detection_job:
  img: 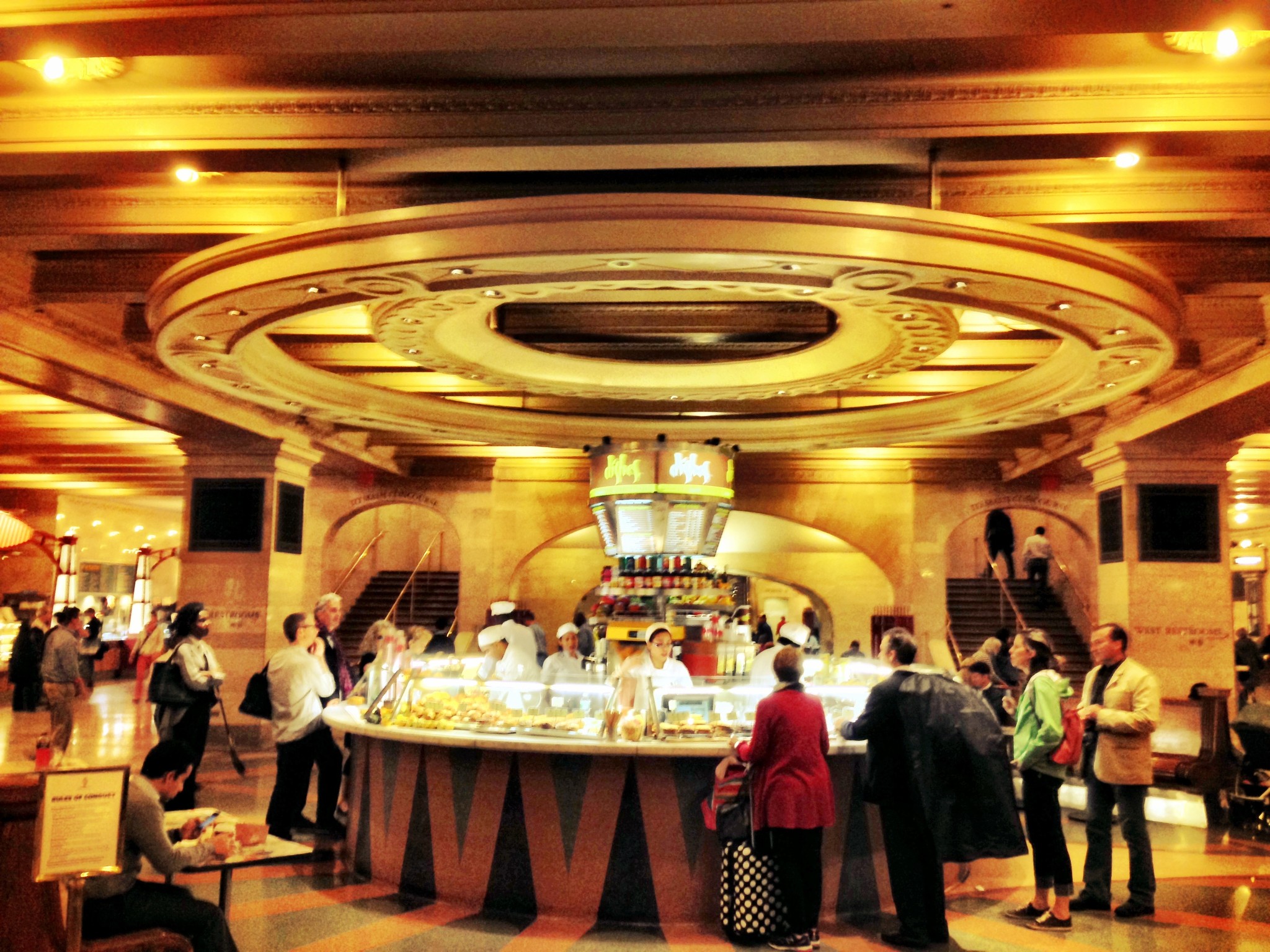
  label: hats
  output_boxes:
[490,600,515,616]
[477,624,505,651]
[556,622,579,639]
[779,622,811,645]
[645,621,671,643]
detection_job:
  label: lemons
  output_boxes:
[348,695,454,730]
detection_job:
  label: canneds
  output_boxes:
[609,556,707,588]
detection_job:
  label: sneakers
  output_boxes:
[810,929,821,946]
[1026,911,1073,930]
[1005,902,1050,918]
[767,932,812,951]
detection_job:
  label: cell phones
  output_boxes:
[197,810,221,829]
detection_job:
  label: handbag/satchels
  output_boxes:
[702,754,749,830]
[78,637,100,654]
[238,662,272,721]
[35,743,53,771]
[145,645,195,706]
[716,796,745,835]
[339,667,352,698]
[1052,698,1085,765]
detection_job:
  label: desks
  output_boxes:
[155,805,320,922]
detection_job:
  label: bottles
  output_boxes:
[624,558,633,587]
[718,563,729,589]
[645,556,661,588]
[634,557,646,588]
[680,557,693,588]
[671,557,680,588]
[35,733,51,770]
[610,558,624,588]
[662,557,671,587]
[693,562,714,589]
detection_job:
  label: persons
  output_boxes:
[981,508,1016,580]
[1188,622,1270,771]
[729,645,836,952]
[627,622,694,711]
[840,640,865,658]
[1022,526,1051,608]
[957,627,1025,745]
[0,592,609,952]
[835,627,950,952]
[1003,629,1075,931]
[1066,622,1161,919]
[724,605,823,684]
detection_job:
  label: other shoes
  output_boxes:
[879,929,948,951]
[1069,896,1110,913]
[1114,898,1155,918]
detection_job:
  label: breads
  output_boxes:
[620,715,754,742]
[418,690,584,732]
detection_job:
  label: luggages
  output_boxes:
[719,766,785,946]
